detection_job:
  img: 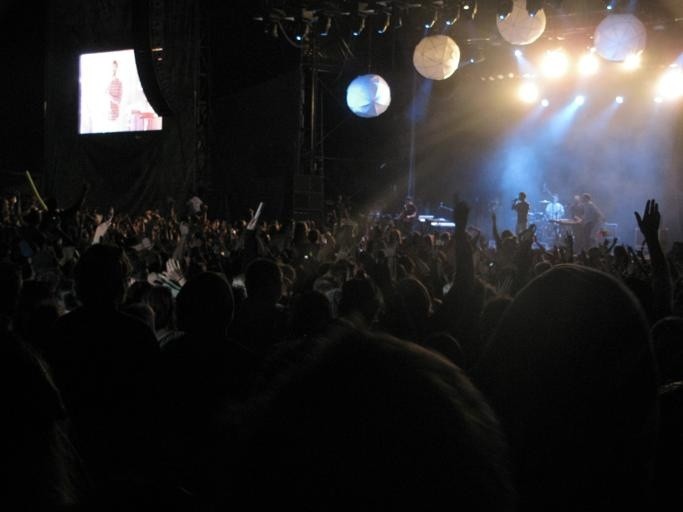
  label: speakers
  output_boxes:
[133,0,186,116]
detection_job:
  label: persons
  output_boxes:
[104,60,121,122]
[1,191,682,510]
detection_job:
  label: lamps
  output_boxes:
[594,12,648,62]
[412,34,460,82]
[346,24,391,118]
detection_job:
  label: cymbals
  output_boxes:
[538,200,551,204]
